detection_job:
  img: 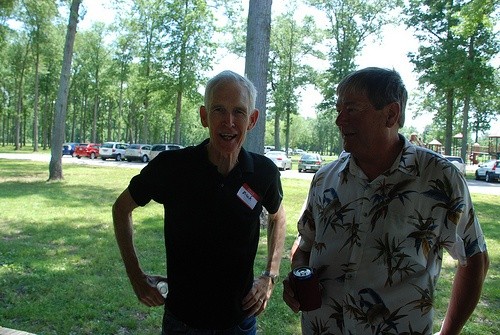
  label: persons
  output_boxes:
[283,67,491,335]
[111,70,287,335]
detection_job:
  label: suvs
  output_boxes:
[443,156,467,177]
[62,141,184,164]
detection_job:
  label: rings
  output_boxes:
[260,300,263,303]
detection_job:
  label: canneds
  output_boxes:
[292,266,313,279]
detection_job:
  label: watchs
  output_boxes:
[262,271,279,283]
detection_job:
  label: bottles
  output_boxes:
[156,282,168,298]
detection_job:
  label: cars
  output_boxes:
[298,154,325,173]
[264,151,293,171]
[475,160,500,182]
[264,146,304,155]
